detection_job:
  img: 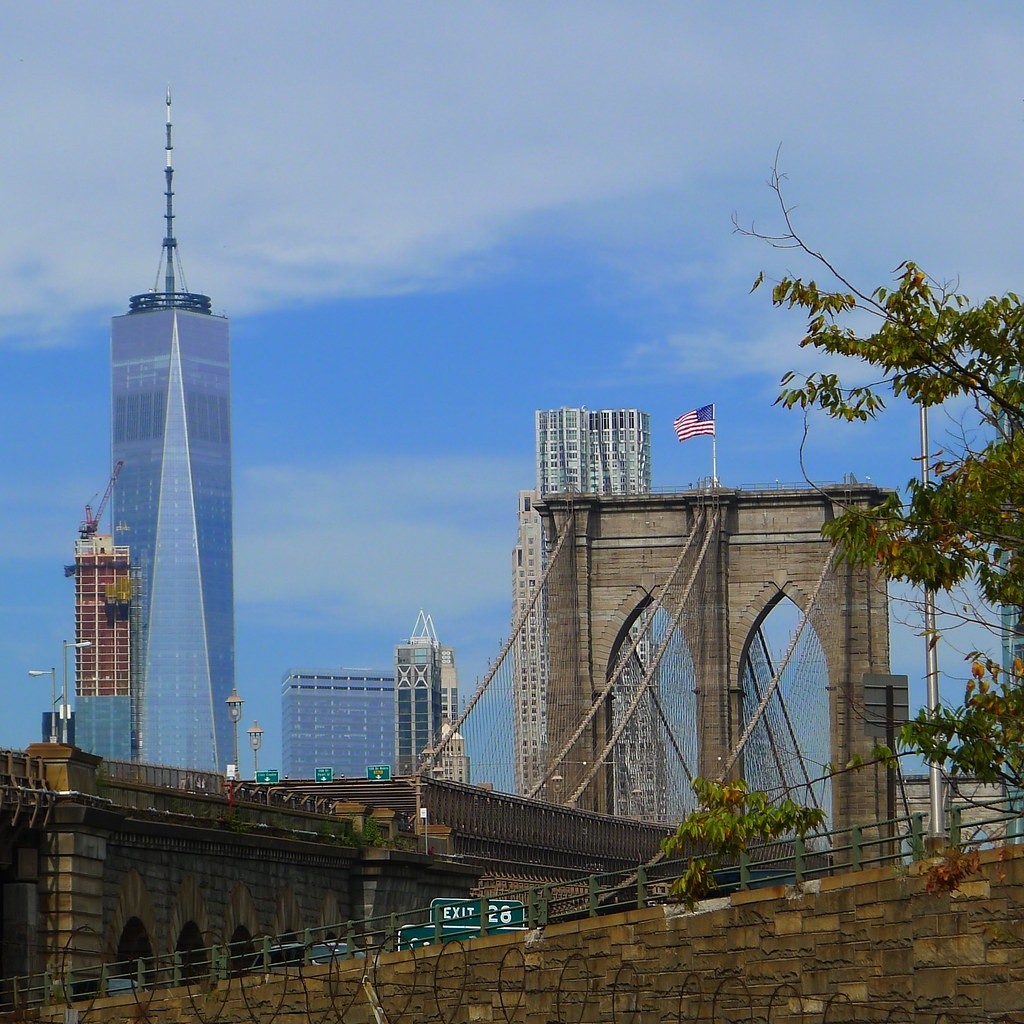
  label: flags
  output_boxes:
[673,403,714,442]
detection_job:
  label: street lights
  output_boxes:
[63,641,93,743]
[28,667,58,743]
[247,721,264,783]
[225,689,245,781]
[552,769,564,805]
